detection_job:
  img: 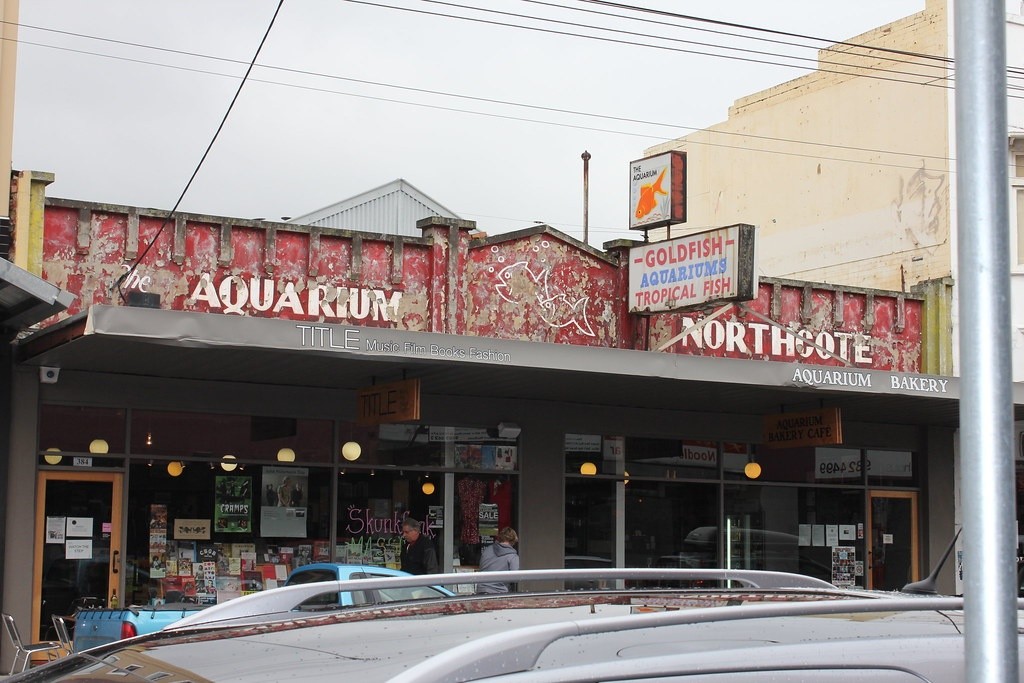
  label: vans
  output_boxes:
[680,526,815,589]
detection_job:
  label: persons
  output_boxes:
[400,519,437,575]
[477,528,520,594]
[205,580,217,594]
[873,528,885,580]
[281,555,290,564]
[197,565,204,579]
[153,556,159,567]
[181,560,190,571]
[266,476,302,507]
[153,541,165,548]
[151,519,166,529]
[299,550,310,559]
[249,579,262,590]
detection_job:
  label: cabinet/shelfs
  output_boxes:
[724,511,765,589]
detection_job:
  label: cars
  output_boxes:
[564,556,624,592]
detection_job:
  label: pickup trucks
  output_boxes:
[74,564,456,655]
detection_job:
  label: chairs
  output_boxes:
[0,612,74,677]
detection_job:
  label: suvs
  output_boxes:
[0,569,1024,683]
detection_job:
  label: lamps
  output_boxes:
[44,447,64,465]
[166,461,185,477]
[220,455,238,471]
[277,448,296,463]
[623,472,630,485]
[580,462,597,476]
[88,439,110,454]
[422,482,435,496]
[744,462,763,480]
[342,441,362,461]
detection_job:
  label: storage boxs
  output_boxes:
[454,444,518,471]
